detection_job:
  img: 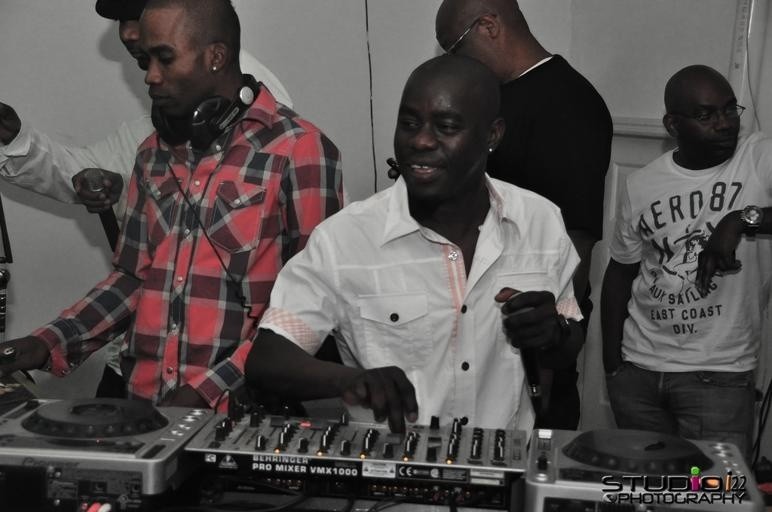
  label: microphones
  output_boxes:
[84,169,121,253]
[507,292,543,398]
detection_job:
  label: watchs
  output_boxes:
[743,205,760,238]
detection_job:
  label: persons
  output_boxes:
[245,54,580,429]
[600,66,772,465]
[0,0,344,416]
[0,0,293,398]
[388,0,613,344]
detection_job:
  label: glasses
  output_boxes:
[667,104,746,124]
[441,13,497,54]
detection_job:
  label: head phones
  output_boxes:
[151,73,261,155]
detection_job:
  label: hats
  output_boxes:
[95,1,147,20]
